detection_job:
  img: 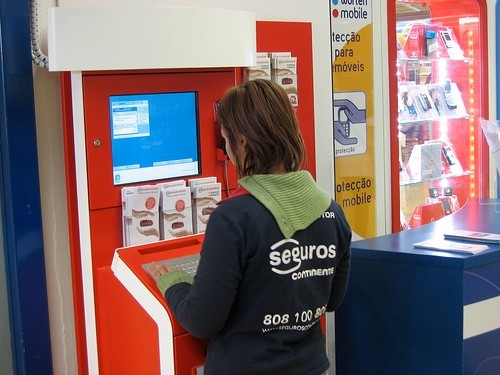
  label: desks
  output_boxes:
[335,196,500,375]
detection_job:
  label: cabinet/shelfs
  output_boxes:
[396,55,475,230]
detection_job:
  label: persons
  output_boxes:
[146,77,353,375]
[402,89,444,120]
